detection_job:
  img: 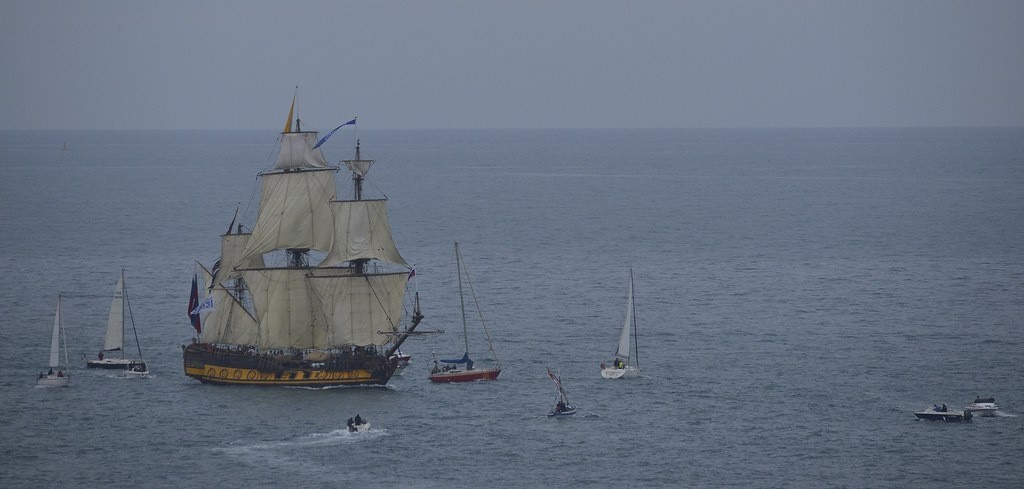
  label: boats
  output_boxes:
[914,405,960,421]
[965,398,998,417]
[347,417,371,435]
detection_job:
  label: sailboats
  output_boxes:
[183,84,423,387]
[600,264,641,379]
[547,366,576,417]
[86,268,146,372]
[35,295,70,386]
[426,241,502,383]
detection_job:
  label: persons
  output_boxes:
[98,350,104,361]
[354,414,362,426]
[347,416,354,431]
[38,369,64,379]
[614,358,623,369]
